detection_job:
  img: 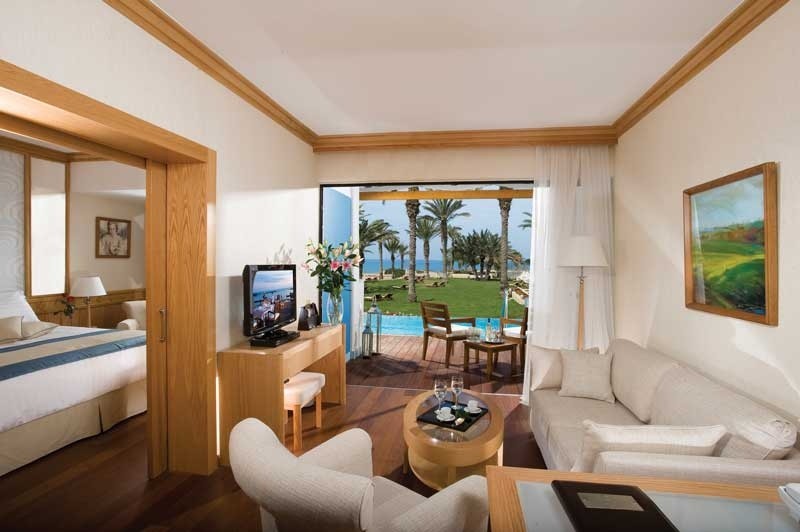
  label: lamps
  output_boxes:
[70,277,108,326]
[557,234,609,350]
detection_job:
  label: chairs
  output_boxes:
[228,417,490,532]
[496,305,528,374]
[420,301,475,367]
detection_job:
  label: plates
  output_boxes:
[464,407,482,414]
[436,414,456,421]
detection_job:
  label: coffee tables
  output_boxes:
[403,387,503,490]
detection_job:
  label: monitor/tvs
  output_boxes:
[243,265,300,347]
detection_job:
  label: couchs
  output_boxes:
[529,338,800,476]
[116,300,147,332]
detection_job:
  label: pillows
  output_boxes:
[570,418,725,472]
[559,351,616,403]
[0,316,25,342]
[22,321,58,339]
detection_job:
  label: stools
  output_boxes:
[285,371,327,450]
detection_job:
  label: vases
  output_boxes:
[327,288,344,326]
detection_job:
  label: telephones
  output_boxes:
[778,483,800,522]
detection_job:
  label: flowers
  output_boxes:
[55,293,76,320]
[303,239,364,292]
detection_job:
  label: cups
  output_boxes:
[438,407,451,418]
[468,400,479,411]
[466,327,482,341]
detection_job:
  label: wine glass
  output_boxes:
[433,378,448,414]
[451,376,463,410]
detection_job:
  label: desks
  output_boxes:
[465,338,517,379]
[217,322,346,467]
[486,465,800,532]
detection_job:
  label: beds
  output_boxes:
[0,291,147,478]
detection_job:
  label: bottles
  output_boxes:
[486,317,492,341]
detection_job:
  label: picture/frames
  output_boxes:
[681,161,781,325]
[95,217,132,261]
